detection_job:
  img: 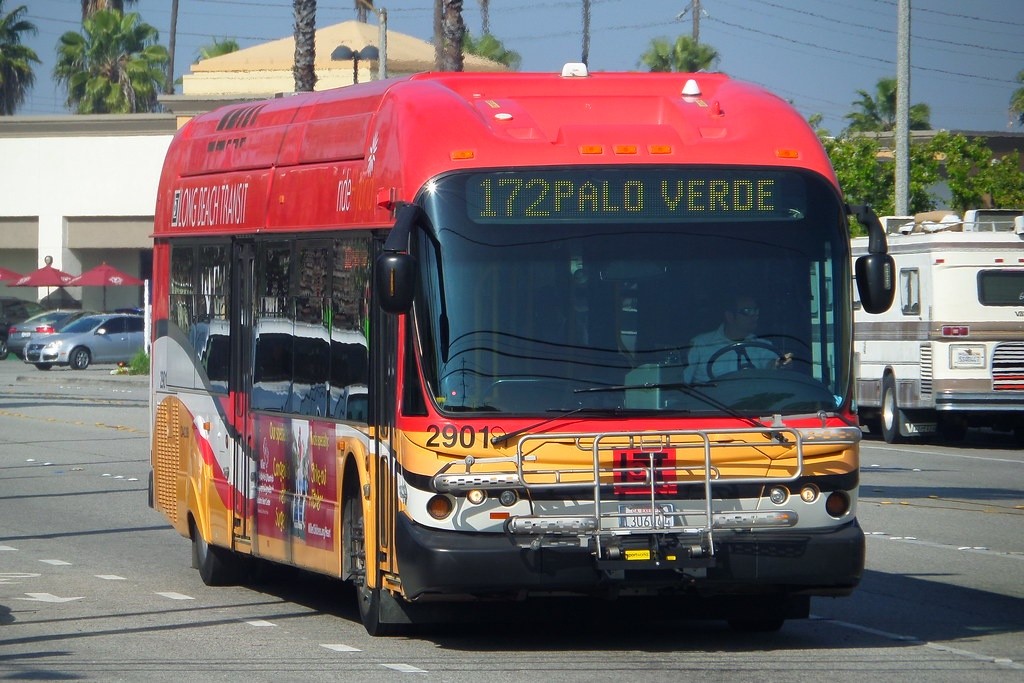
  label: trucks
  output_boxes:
[843,207,1024,445]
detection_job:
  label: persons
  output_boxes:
[683,286,795,385]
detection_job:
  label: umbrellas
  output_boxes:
[0,255,144,308]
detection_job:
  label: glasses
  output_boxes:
[733,308,760,317]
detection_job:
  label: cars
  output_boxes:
[22,313,147,373]
[8,309,100,361]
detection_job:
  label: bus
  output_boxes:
[146,61,898,642]
